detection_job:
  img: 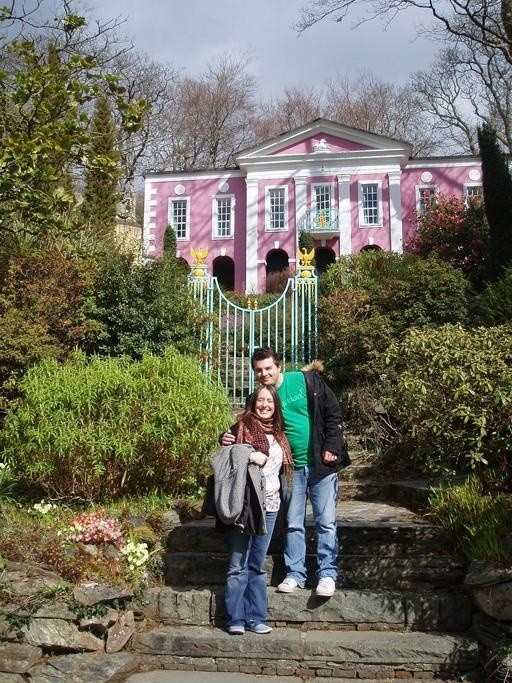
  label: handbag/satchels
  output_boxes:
[201,474,217,515]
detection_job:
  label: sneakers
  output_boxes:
[277,577,299,592]
[315,577,335,596]
[228,624,273,634]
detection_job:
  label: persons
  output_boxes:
[219,348,352,597]
[223,384,293,634]
